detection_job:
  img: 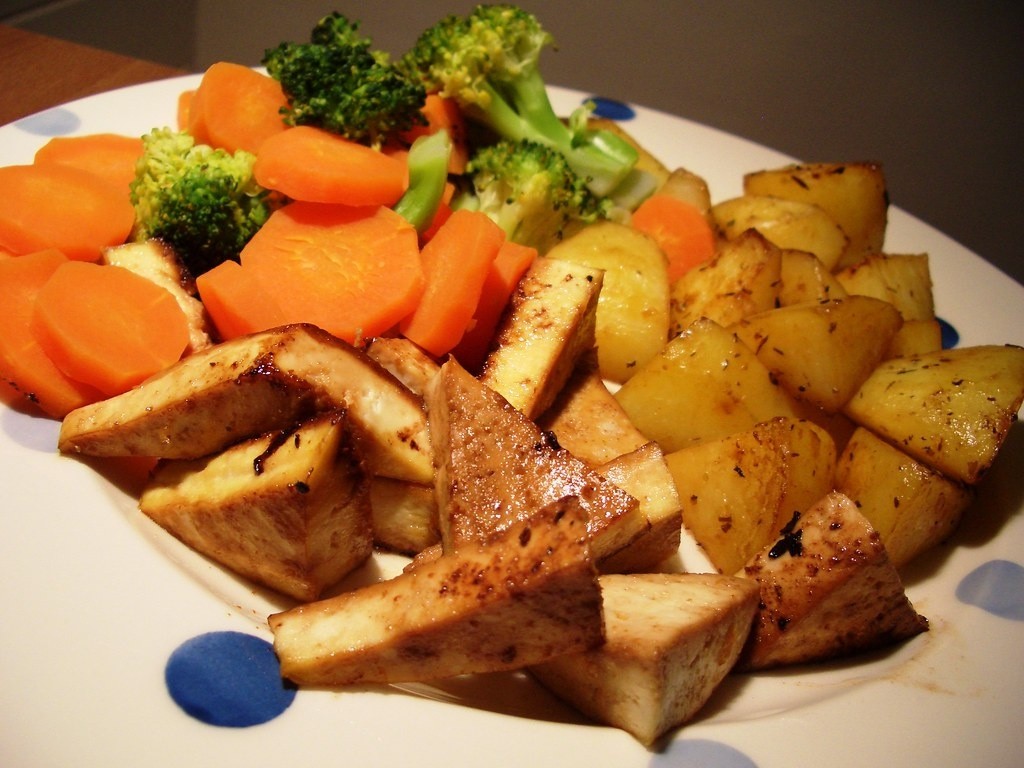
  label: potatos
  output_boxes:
[545,158,1024,575]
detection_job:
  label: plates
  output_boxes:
[0,66,1023,768]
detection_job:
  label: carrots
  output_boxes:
[0,61,713,414]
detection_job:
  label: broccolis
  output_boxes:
[127,3,641,273]
